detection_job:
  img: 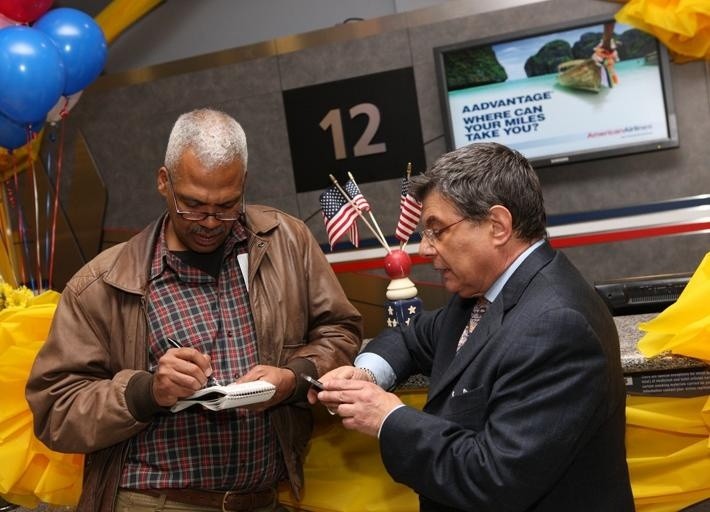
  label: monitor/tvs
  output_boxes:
[432,12,680,171]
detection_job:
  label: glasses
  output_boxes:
[167,171,248,223]
[417,209,485,240]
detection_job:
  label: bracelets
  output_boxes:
[358,367,376,386]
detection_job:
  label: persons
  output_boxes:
[308,140,636,512]
[24,107,364,512]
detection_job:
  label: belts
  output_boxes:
[128,485,280,512]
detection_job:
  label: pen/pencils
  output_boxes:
[167,338,223,387]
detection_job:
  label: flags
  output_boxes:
[345,178,371,249]
[394,176,422,244]
[319,184,362,252]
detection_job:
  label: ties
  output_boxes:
[456,301,488,352]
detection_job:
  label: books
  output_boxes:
[169,378,277,414]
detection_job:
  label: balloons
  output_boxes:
[0,0,110,156]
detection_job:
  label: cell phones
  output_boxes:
[299,372,324,391]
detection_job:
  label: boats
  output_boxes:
[645,52,658,65]
[557,23,617,94]
